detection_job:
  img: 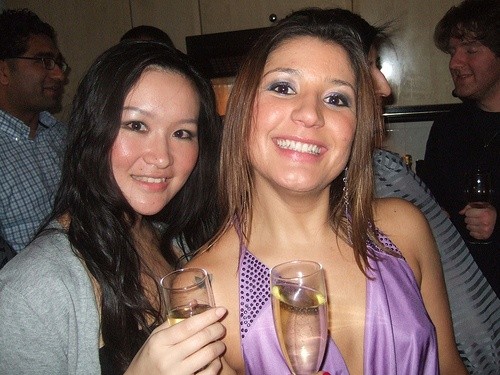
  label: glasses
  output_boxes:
[0,53,68,73]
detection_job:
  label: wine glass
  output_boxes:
[460,166,497,246]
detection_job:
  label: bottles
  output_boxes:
[402,153,413,167]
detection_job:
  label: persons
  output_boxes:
[331,8,500,375]
[423,0,500,301]
[0,40,228,375]
[0,8,68,269]
[171,16,466,375]
[120,25,176,51]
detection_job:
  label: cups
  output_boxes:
[159,266,216,328]
[269,258,331,375]
